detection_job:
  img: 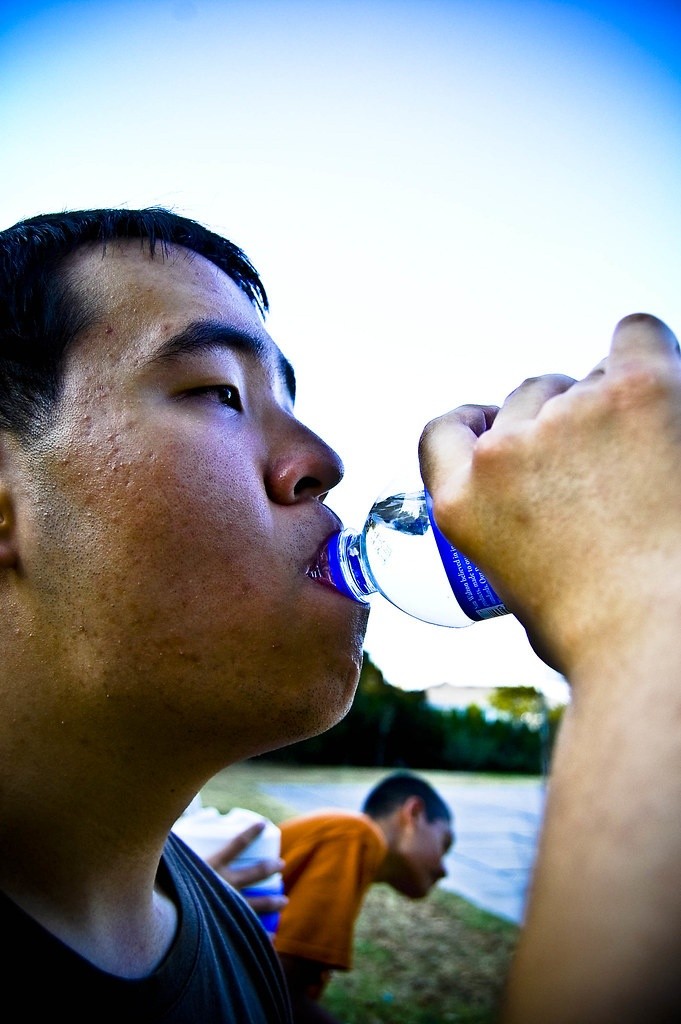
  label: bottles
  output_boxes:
[306,484,514,629]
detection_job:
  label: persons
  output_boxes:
[210,823,289,914]
[1,208,681,1024]
[273,772,454,1006]
